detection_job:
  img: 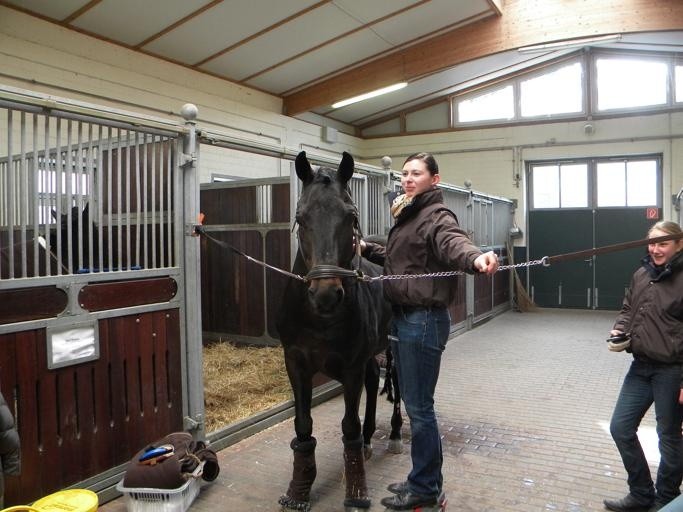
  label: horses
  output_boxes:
[50,201,124,275]
[276,151,403,511]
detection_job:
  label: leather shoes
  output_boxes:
[381,490,440,510]
[603,490,658,512]
[386,478,443,495]
[647,488,682,512]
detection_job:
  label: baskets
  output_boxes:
[115,456,206,512]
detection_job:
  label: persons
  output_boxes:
[348,150,501,509]
[600,220,683,511]
